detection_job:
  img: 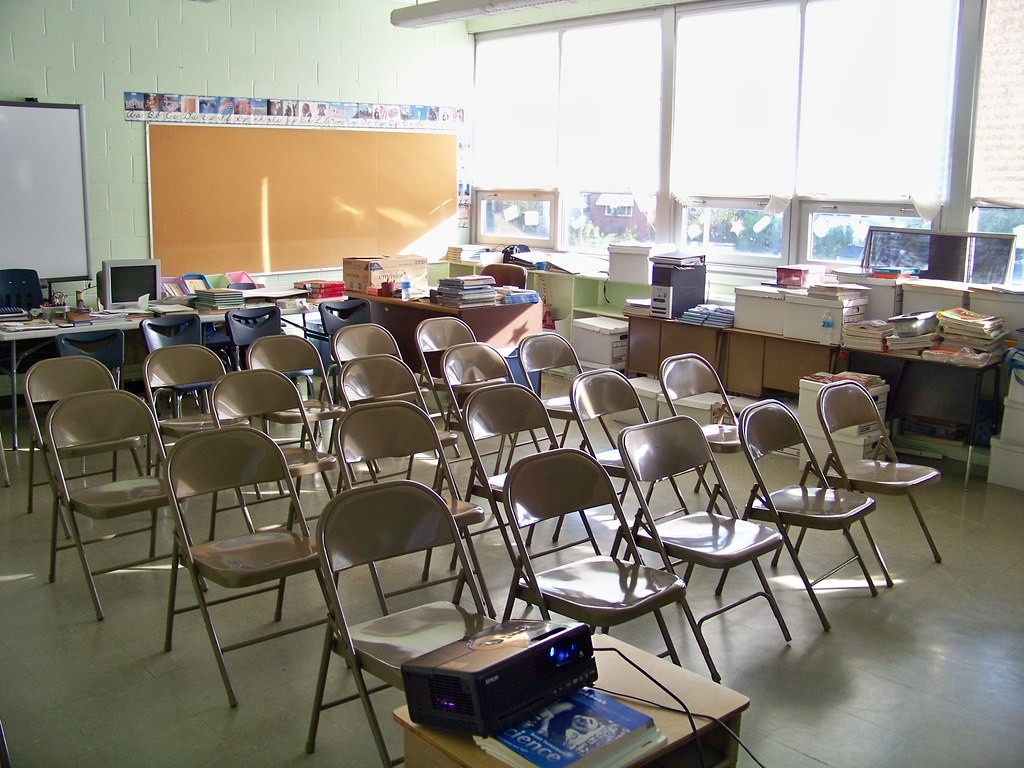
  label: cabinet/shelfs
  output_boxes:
[720,327,839,399]
[627,312,720,376]
[448,260,652,376]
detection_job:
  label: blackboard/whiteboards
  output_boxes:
[0,98,93,288]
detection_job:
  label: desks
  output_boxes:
[393,635,747,767]
[845,347,1003,493]
[0,289,543,450]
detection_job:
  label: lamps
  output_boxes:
[390,0,553,31]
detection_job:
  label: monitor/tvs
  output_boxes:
[95,260,162,311]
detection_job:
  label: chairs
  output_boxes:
[25,296,946,767]
[481,263,528,291]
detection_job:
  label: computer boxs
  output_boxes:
[650,267,706,320]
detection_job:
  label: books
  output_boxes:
[65,243,1010,352]
[474,686,668,768]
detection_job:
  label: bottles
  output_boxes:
[819,310,833,345]
[401,271,411,301]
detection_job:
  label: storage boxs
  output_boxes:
[987,366,1024,491]
[572,316,625,362]
[734,286,785,337]
[769,398,799,459]
[797,378,890,436]
[341,255,427,292]
[799,430,890,481]
[713,397,759,444]
[656,389,732,435]
[785,294,867,342]
[611,377,662,426]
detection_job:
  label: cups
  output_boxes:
[381,282,395,297]
